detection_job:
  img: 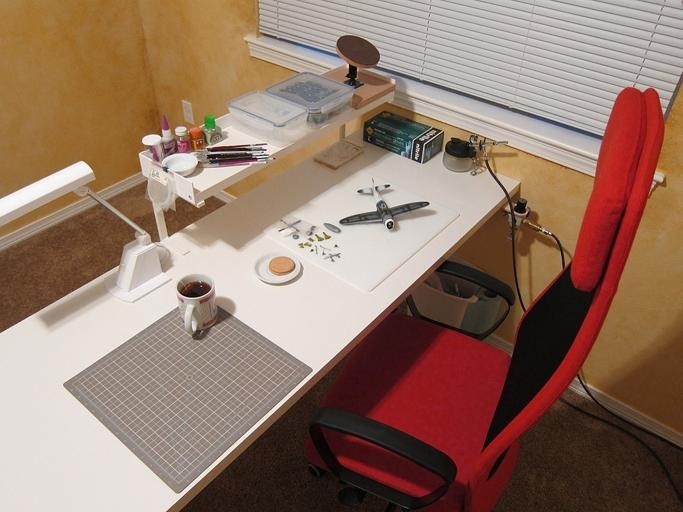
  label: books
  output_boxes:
[321,64,395,109]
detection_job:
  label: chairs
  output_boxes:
[307,86,663,511]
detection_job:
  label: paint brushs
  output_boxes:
[207,143,276,166]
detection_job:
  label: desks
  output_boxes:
[0,65,523,510]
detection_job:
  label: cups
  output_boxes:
[174,273,218,334]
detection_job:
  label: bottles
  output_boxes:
[443,137,476,174]
[142,113,223,164]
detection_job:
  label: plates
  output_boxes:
[253,252,303,285]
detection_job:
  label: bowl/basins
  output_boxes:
[162,153,198,176]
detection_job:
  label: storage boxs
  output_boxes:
[262,71,355,132]
[225,90,307,139]
